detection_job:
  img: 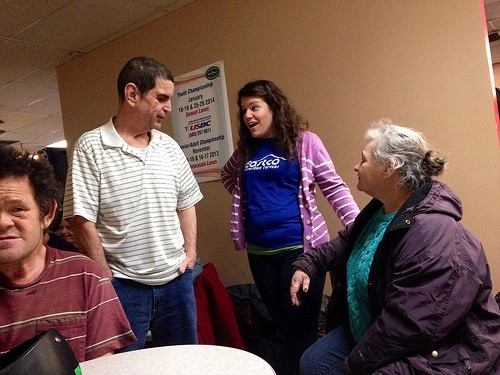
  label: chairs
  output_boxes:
[193,262,218,328]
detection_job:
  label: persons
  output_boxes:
[63,56,204,352]
[291,119,500,375]
[220,80,363,375]
[0,144,140,363]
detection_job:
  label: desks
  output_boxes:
[78,344,276,375]
